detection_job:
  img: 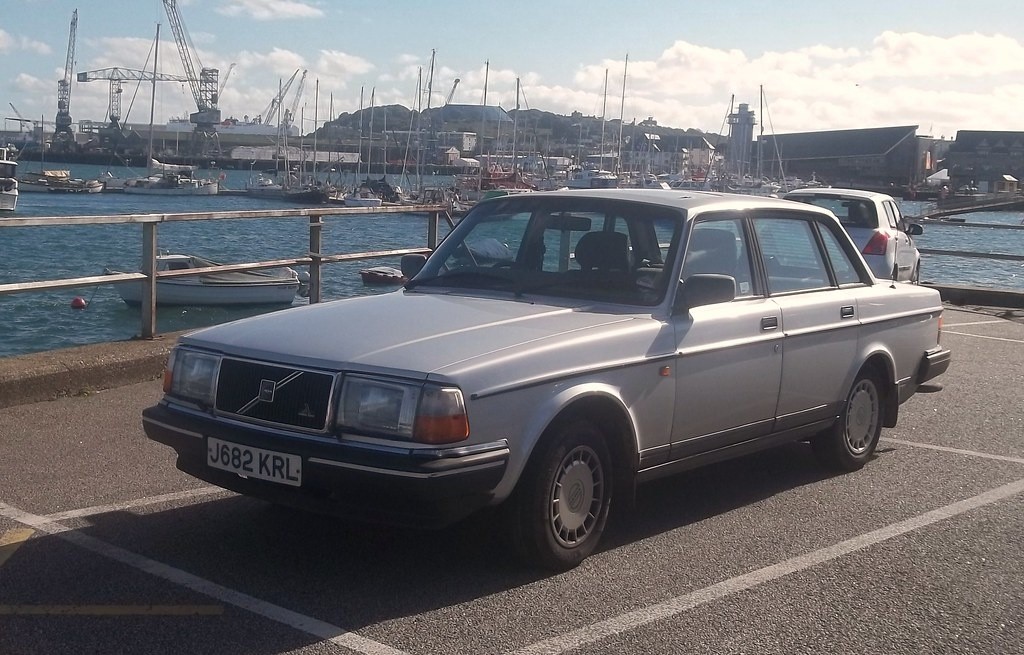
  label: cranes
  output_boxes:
[283,69,308,125]
[49,8,78,153]
[76,67,195,152]
[161,0,223,157]
[257,68,301,125]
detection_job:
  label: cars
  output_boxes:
[765,187,924,286]
[139,187,953,574]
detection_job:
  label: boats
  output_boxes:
[101,254,301,305]
[0,49,821,212]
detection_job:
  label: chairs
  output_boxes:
[574,231,632,291]
[682,228,736,280]
[848,201,874,225]
